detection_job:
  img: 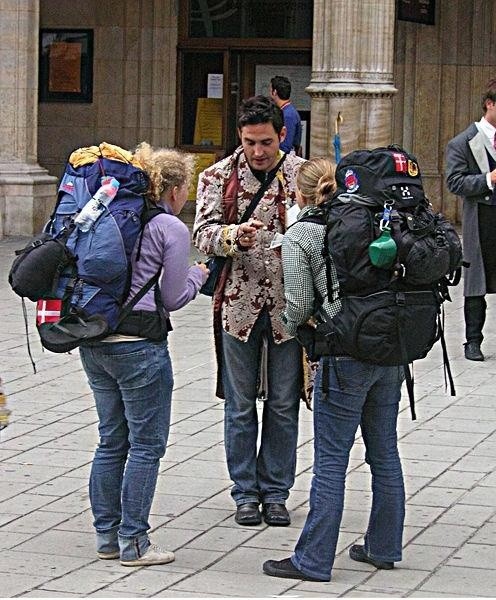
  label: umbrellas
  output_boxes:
[333,116,343,164]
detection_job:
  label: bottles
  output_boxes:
[72,179,120,233]
[369,227,397,270]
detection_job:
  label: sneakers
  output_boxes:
[97,543,175,566]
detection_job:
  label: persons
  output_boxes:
[192,95,309,527]
[268,76,302,155]
[263,157,406,582]
[78,142,211,567]
[445,79,496,361]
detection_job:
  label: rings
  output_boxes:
[245,236,249,242]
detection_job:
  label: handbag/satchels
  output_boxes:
[199,252,228,295]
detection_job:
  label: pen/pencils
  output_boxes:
[233,224,254,245]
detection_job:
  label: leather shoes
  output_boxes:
[465,341,484,361]
[235,501,290,526]
[263,558,330,581]
[349,545,394,569]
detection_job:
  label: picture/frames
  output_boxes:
[37,27,95,104]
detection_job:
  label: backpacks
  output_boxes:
[303,144,470,419]
[8,142,165,374]
